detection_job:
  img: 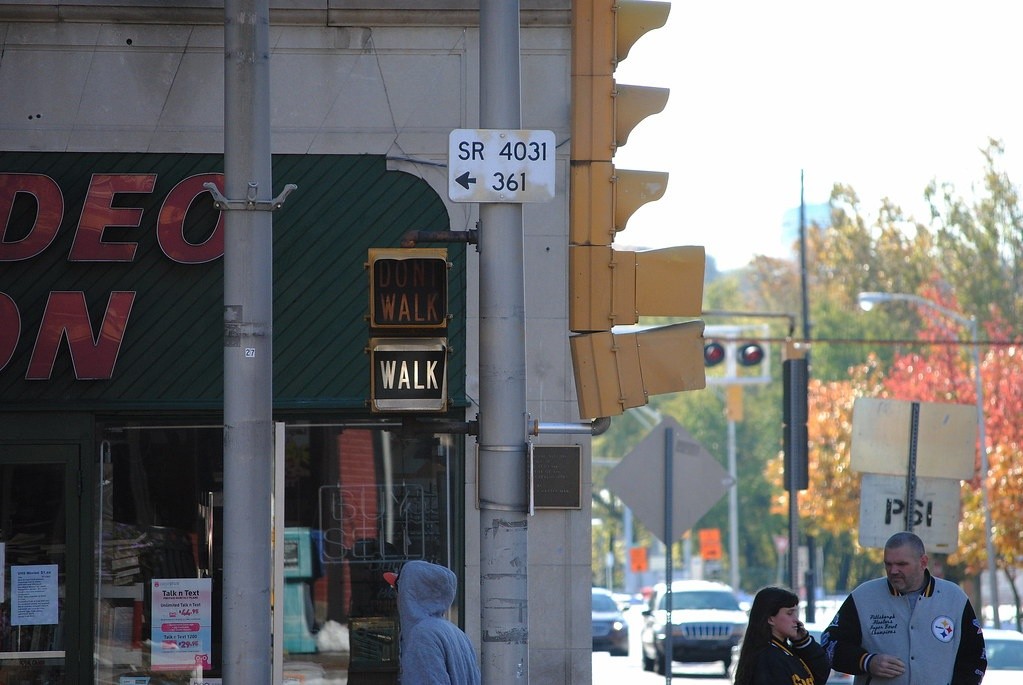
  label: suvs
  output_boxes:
[640,580,750,675]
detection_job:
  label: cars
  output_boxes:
[980,628,1023,685]
[728,625,854,685]
[590,587,630,656]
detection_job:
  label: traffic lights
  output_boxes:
[702,325,771,382]
[567,0,708,420]
[363,247,449,413]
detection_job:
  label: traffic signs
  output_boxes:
[448,128,557,204]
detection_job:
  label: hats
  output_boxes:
[383,560,406,594]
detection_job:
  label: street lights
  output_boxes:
[857,292,1000,630]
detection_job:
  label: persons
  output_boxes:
[733,587,831,685]
[821,532,988,685]
[383,560,482,685]
